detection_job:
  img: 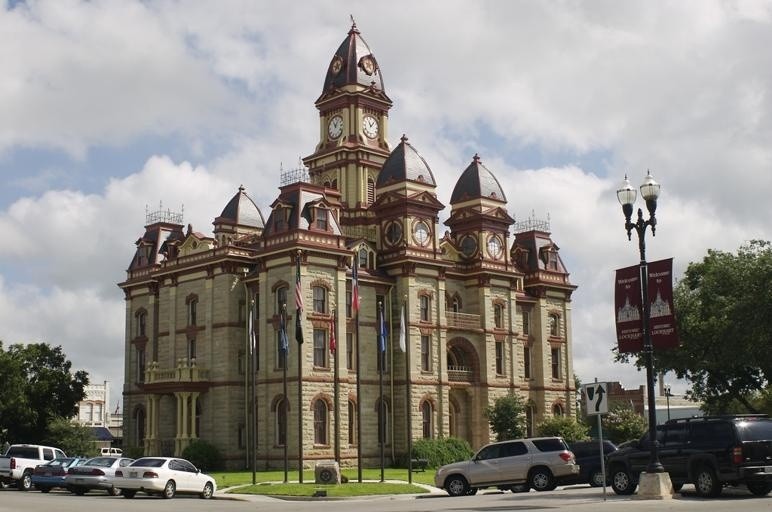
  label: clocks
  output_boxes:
[363,114,380,139]
[327,115,343,140]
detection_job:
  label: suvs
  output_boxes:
[605,411,772,501]
[511,438,620,493]
[436,436,581,496]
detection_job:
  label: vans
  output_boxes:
[96,447,124,459]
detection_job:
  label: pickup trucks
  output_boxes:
[0,444,68,492]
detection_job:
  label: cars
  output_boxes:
[112,456,217,498]
[65,454,135,496]
[30,456,87,495]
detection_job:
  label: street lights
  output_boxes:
[616,168,669,475]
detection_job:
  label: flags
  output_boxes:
[247,308,257,355]
[351,253,360,314]
[293,259,304,316]
[399,305,406,352]
[278,308,289,352]
[329,312,336,354]
[379,312,386,354]
